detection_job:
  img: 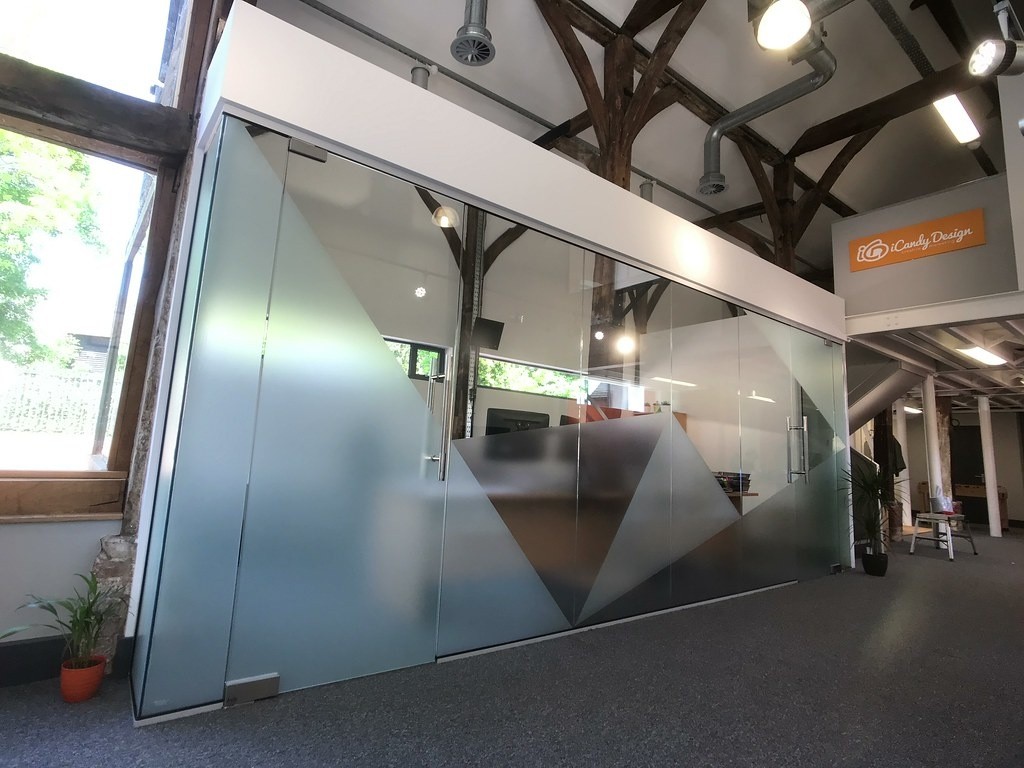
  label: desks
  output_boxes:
[910,513,977,561]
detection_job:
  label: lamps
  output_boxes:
[968,0,1024,78]
[754,0,811,50]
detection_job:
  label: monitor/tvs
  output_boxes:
[470,317,505,350]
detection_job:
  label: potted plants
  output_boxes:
[0,573,134,703]
[836,455,912,577]
